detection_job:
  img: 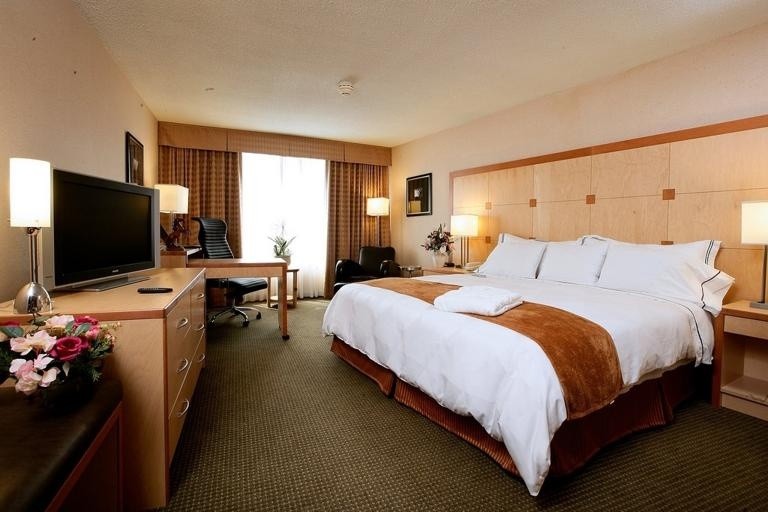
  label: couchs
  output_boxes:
[333,246,401,294]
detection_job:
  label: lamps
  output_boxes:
[741,200,768,310]
[154,184,189,244]
[9,158,51,313]
[450,215,478,267]
[366,197,390,247]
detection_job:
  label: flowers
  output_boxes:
[420,223,456,256]
[0,314,120,395]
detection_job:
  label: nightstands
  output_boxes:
[712,300,768,421]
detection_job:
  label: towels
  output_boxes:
[434,285,523,316]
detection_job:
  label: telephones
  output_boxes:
[465,262,480,271]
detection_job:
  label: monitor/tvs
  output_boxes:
[37,167,160,292]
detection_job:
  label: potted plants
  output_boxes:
[268,225,297,266]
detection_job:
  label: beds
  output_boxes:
[322,271,714,496]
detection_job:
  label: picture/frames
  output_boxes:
[406,173,432,217]
[125,131,144,185]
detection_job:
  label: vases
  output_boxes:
[433,250,446,268]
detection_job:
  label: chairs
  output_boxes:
[191,217,268,327]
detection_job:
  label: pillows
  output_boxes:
[474,232,721,304]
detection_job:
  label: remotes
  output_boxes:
[137,287,173,293]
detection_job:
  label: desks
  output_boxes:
[0,268,207,506]
[1,378,129,511]
[187,258,289,340]
